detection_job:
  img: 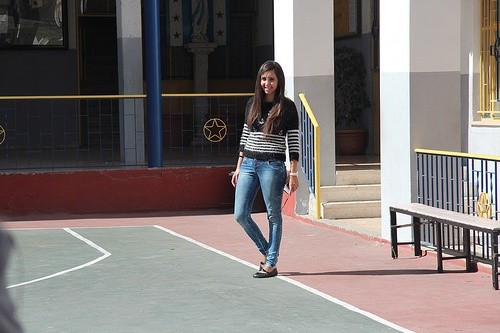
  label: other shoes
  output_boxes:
[252,259,279,278]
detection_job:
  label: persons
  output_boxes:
[231,61,300,278]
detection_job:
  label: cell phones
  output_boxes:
[284,183,290,196]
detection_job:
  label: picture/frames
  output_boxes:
[0,0,69,51]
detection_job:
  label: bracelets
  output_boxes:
[238,156,243,158]
[288,172,298,177]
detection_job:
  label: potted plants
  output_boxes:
[334,44,372,156]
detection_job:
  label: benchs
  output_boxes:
[389,203,500,290]
